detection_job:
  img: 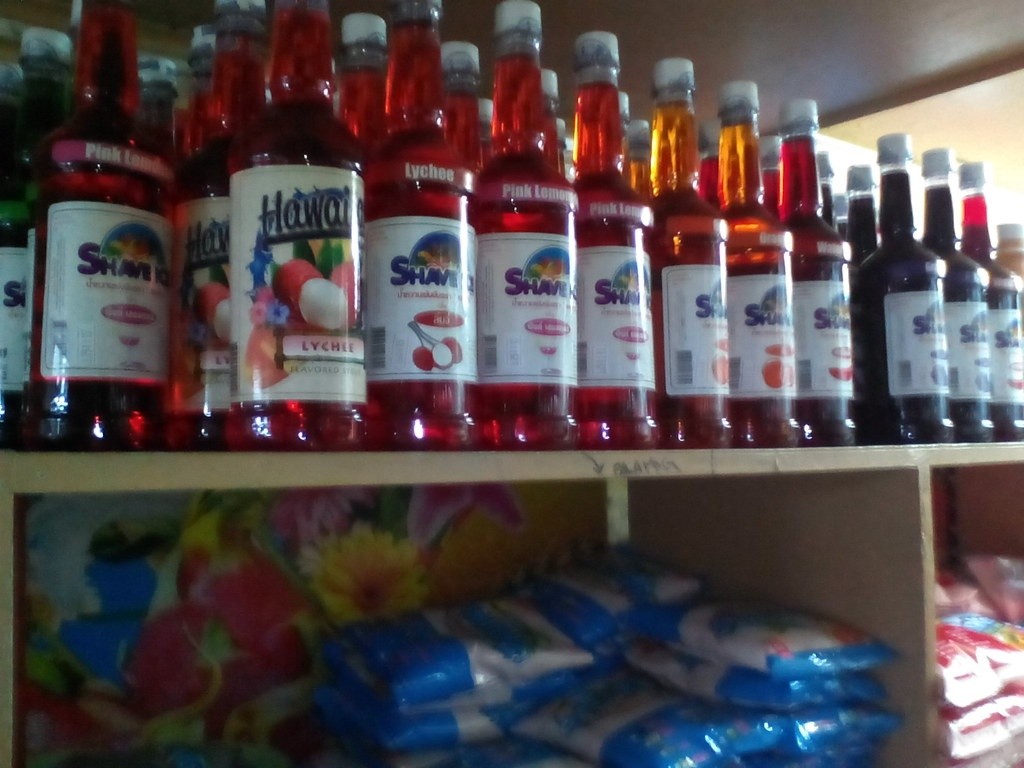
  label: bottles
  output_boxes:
[0,0,1023,449]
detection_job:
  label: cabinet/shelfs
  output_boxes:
[0,441,1024,768]
